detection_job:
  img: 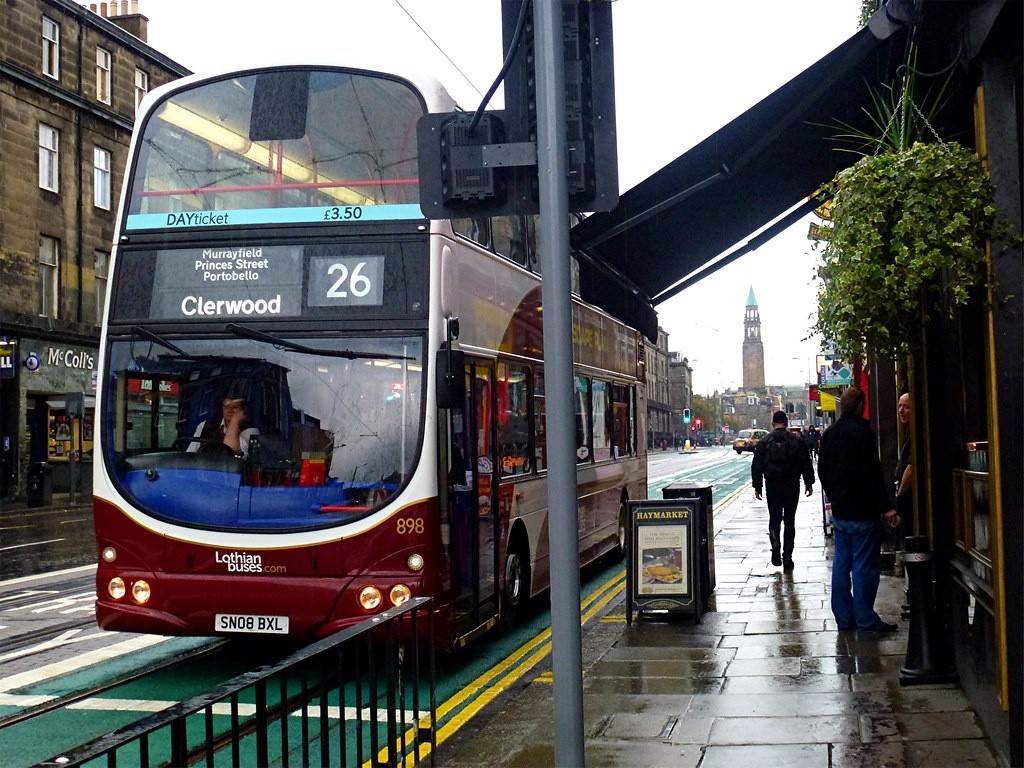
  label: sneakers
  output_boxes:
[784,558,794,570]
[771,543,781,566]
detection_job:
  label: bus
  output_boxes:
[93,58,649,656]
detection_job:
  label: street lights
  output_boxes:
[791,357,817,426]
[706,372,735,446]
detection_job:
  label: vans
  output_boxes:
[733,429,770,454]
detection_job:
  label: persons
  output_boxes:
[751,411,816,571]
[186,388,264,483]
[645,417,829,459]
[886,391,945,615]
[816,386,899,636]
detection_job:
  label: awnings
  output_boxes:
[565,0,946,348]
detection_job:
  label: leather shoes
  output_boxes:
[901,611,911,619]
[901,604,911,610]
[839,622,899,632]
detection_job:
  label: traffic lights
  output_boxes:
[696,418,702,430]
[691,425,696,431]
[683,409,691,424]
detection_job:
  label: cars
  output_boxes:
[786,426,824,448]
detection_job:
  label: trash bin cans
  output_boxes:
[26,462,53,508]
[661,480,716,597]
